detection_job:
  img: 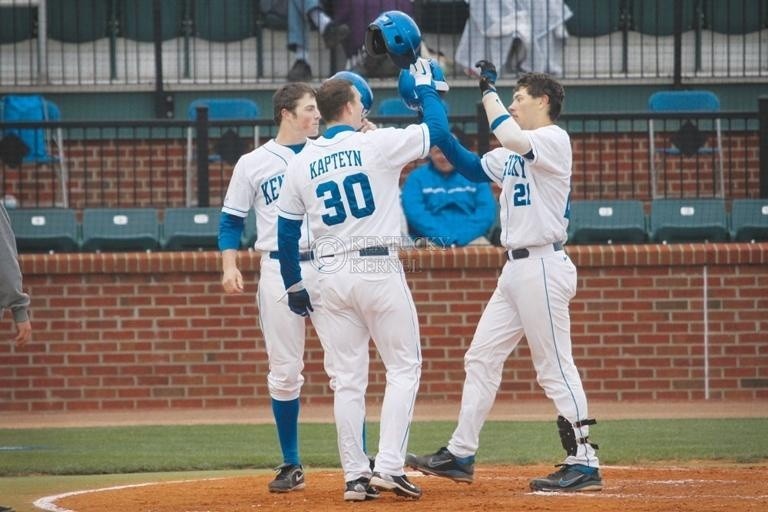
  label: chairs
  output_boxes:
[643,84,727,198]
[4,196,766,254]
[180,97,262,209]
[0,0,768,47]
[0,92,72,207]
[374,94,449,189]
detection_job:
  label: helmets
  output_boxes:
[398,55,449,112]
[363,9,422,63]
[330,71,373,111]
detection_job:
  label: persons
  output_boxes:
[401,59,604,491]
[216,83,368,492]
[261,0,350,81]
[275,60,449,500]
[401,131,498,246]
[0,198,33,349]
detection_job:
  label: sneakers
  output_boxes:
[287,59,311,83]
[528,463,601,492]
[322,21,349,49]
[404,447,474,483]
[368,468,424,502]
[344,476,380,503]
[269,462,307,494]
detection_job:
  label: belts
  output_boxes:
[269,251,317,261]
[321,245,387,257]
[504,241,564,260]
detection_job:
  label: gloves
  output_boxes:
[409,58,433,87]
[476,59,496,93]
[287,289,314,318]
